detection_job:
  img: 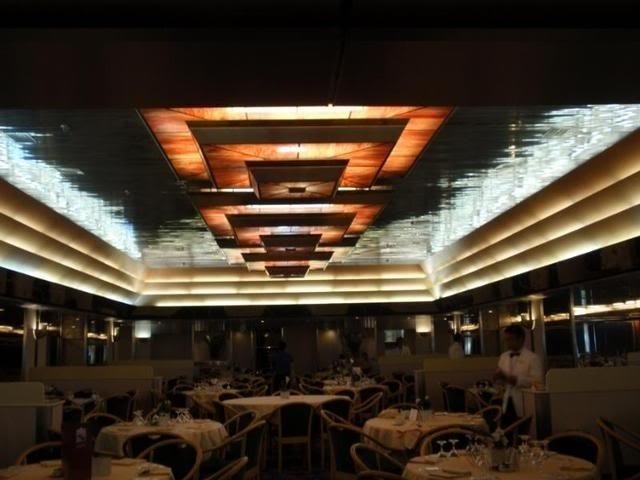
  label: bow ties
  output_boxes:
[509,352,520,358]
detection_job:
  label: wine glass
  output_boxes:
[437,435,550,469]
[192,378,232,393]
[133,408,194,427]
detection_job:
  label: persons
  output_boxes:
[498,325,543,447]
[272,341,295,391]
[394,337,412,356]
[450,334,464,359]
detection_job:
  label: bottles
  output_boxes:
[414,394,432,422]
[474,445,490,480]
[472,379,489,393]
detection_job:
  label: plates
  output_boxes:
[116,421,133,431]
[379,412,486,425]
[424,456,464,477]
